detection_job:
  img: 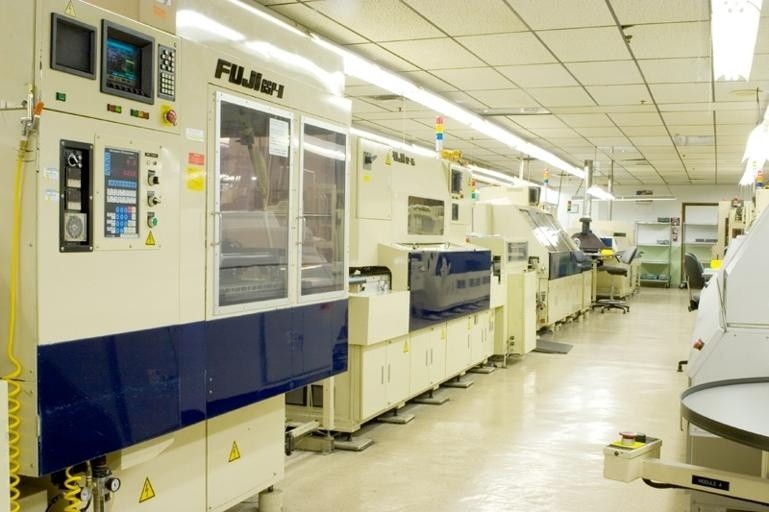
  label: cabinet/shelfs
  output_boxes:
[207,80,354,319]
[632,218,674,290]
[715,187,769,264]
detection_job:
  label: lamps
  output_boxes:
[705,0,764,82]
[566,169,679,202]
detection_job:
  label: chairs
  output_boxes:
[591,244,639,314]
[683,250,710,313]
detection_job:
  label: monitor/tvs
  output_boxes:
[106,35,141,89]
